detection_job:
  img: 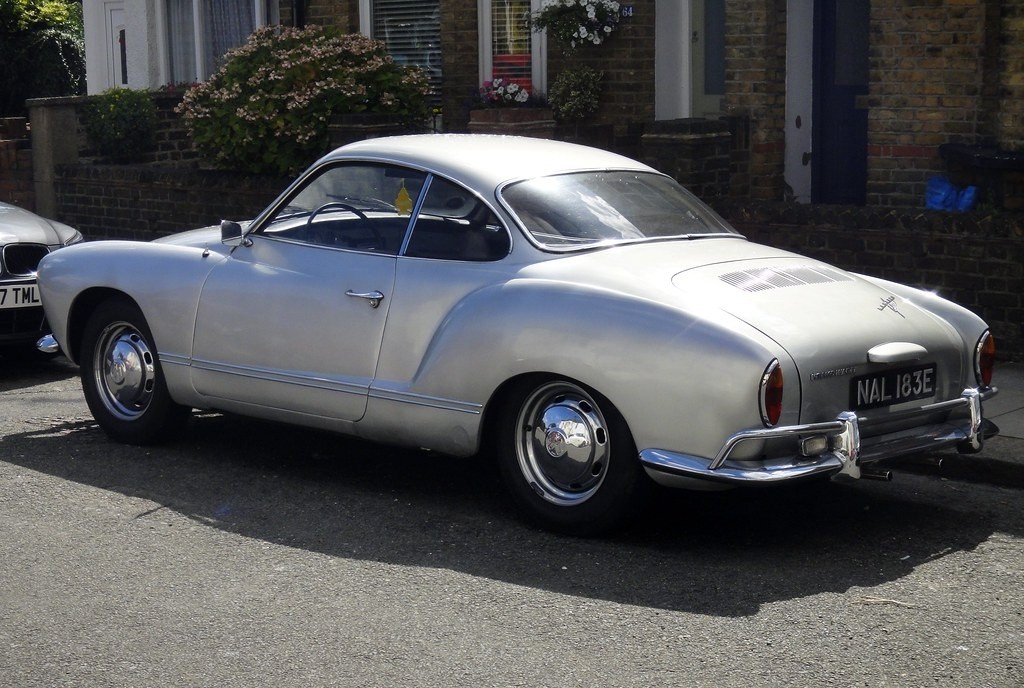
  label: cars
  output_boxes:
[38,134,998,535]
[0,200,85,344]
